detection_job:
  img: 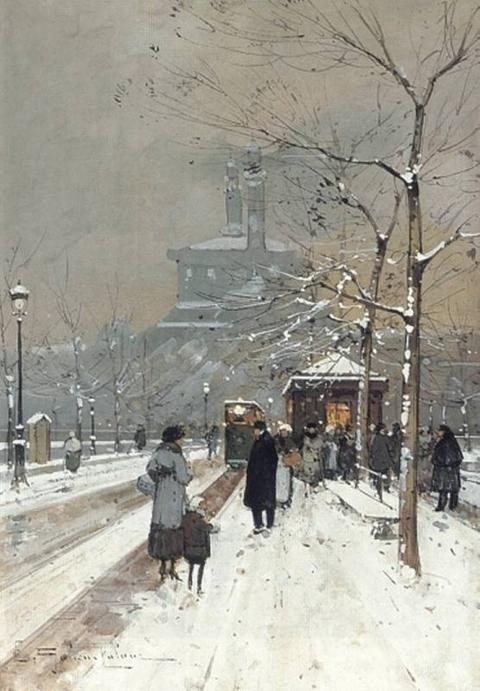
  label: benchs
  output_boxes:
[327,463,401,541]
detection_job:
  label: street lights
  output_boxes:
[8,280,29,486]
[266,395,273,425]
[202,382,210,435]
[87,397,96,457]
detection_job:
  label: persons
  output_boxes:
[430,424,464,512]
[63,431,83,475]
[135,424,147,453]
[242,422,279,535]
[178,496,220,594]
[272,421,439,511]
[147,427,196,581]
[205,422,220,461]
